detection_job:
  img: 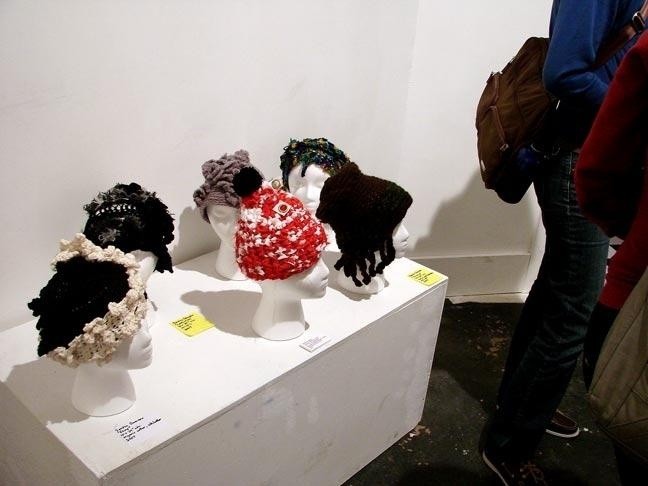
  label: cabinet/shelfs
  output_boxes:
[0,248,450,485]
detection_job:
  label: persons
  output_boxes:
[572,29,646,485]
[228,166,330,342]
[278,137,351,245]
[477,0,646,485]
[26,237,153,418]
[190,147,267,281]
[315,160,414,295]
[81,178,174,314]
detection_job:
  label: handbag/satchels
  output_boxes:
[476,37,557,203]
[587,267,648,463]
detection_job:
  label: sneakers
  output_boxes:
[483,444,546,486]
[545,408,580,438]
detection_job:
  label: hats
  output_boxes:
[28,234,149,367]
[192,138,412,287]
[82,183,175,273]
